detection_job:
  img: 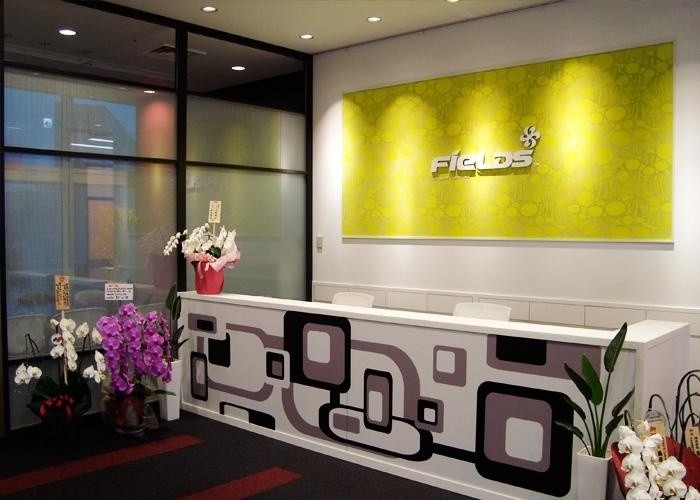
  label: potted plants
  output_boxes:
[553,323,635,500]
[157,284,190,421]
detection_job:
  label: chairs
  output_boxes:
[331,292,375,308]
[452,302,512,321]
[7,287,170,355]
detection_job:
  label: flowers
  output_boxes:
[14,319,107,414]
[616,420,700,500]
[96,304,174,398]
[162,224,241,270]
[137,225,176,257]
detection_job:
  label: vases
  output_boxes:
[151,251,177,287]
[45,411,91,460]
[107,382,148,435]
[189,260,226,293]
